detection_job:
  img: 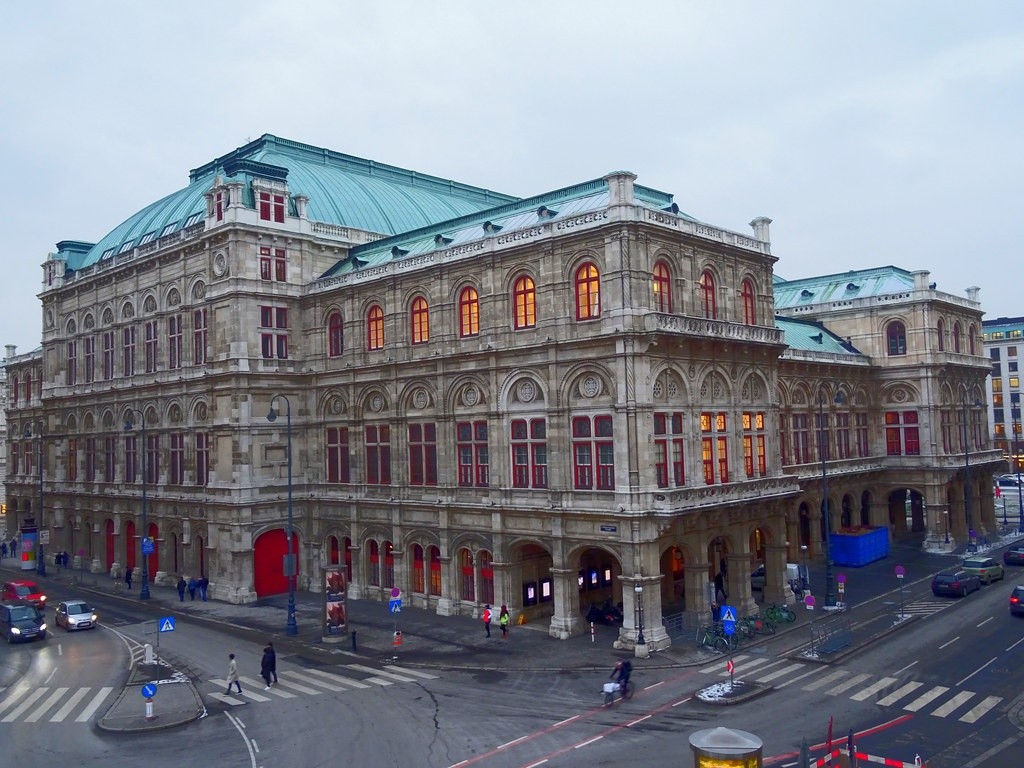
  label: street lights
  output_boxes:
[634,583,645,644]
[962,389,983,552]
[266,395,298,635]
[24,421,45,575]
[944,510,950,544]
[819,379,848,606]
[123,409,150,601]
[1002,494,1008,524]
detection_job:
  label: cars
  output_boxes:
[55,600,97,632]
[1010,585,1024,618]
[0,578,46,609]
[992,473,1024,486]
[931,568,981,597]
[962,557,1005,584]
[1003,546,1024,566]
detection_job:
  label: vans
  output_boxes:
[0,599,46,643]
[751,563,809,590]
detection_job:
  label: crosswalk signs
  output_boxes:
[159,616,176,632]
[722,606,737,622]
[390,601,402,613]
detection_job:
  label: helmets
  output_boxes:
[615,662,624,668]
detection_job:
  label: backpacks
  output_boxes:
[622,662,634,672]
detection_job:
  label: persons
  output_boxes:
[500,605,509,637]
[187,576,199,601]
[125,566,133,590]
[2,542,8,559]
[714,571,728,603]
[223,654,243,697]
[995,486,1001,498]
[55,551,70,572]
[199,575,209,602]
[177,577,186,602]
[483,604,492,638]
[968,528,977,545]
[609,661,631,700]
[588,599,621,625]
[789,575,808,598]
[10,539,17,558]
[260,642,278,691]
[712,603,722,637]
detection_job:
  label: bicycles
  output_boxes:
[600,680,634,709]
[696,612,777,652]
[766,602,797,622]
[790,576,816,600]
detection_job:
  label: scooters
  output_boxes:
[585,594,623,626]
